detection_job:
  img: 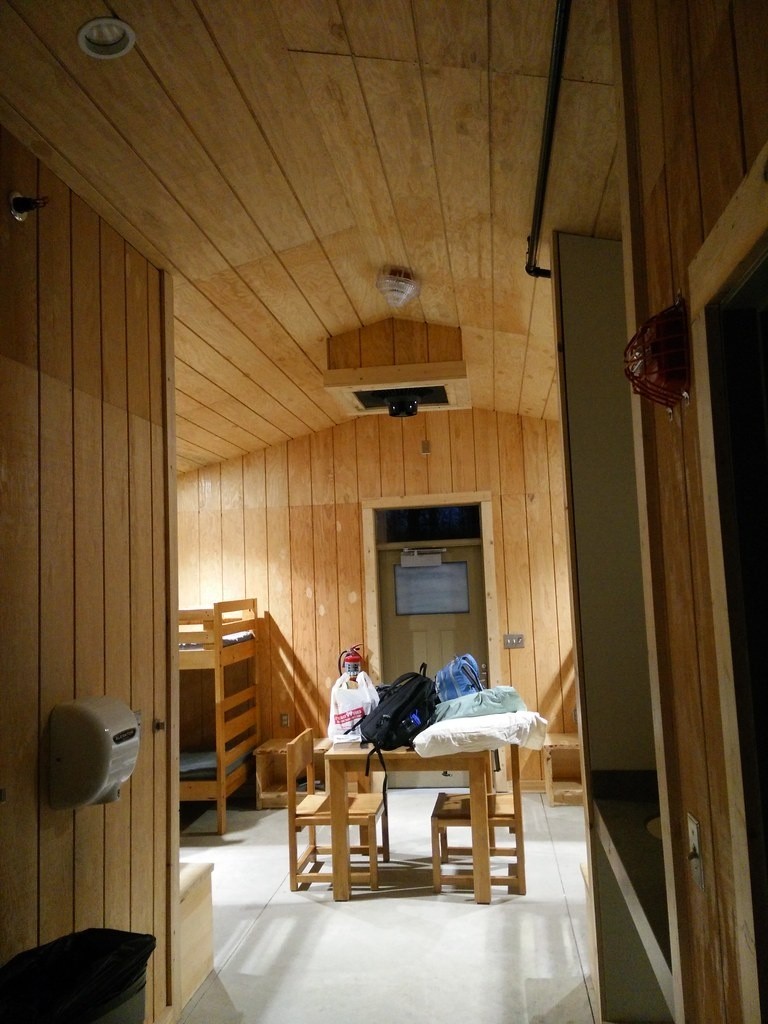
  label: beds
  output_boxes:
[177,598,264,838]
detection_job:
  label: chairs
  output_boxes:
[283,727,529,895]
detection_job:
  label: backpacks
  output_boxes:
[342,662,440,816]
[434,653,483,703]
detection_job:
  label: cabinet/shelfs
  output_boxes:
[543,731,583,806]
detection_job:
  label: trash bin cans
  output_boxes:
[1,927,157,1024]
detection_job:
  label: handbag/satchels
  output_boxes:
[327,672,380,742]
[411,711,548,758]
[431,685,526,722]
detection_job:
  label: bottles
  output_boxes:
[392,715,421,739]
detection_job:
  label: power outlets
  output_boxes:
[280,713,290,728]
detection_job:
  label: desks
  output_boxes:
[324,740,496,905]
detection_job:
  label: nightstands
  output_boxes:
[252,737,373,810]
